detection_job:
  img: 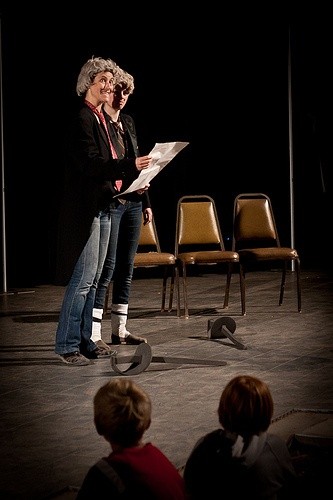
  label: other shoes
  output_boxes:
[94,339,112,351]
[111,331,148,345]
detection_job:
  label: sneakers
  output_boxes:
[54,351,91,366]
[81,346,117,359]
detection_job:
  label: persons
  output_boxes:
[54,56,153,366]
[76,377,182,500]
[184,375,301,500]
[89,72,151,359]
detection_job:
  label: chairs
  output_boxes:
[103,212,182,320]
[227,193,303,316]
[167,195,244,319]
